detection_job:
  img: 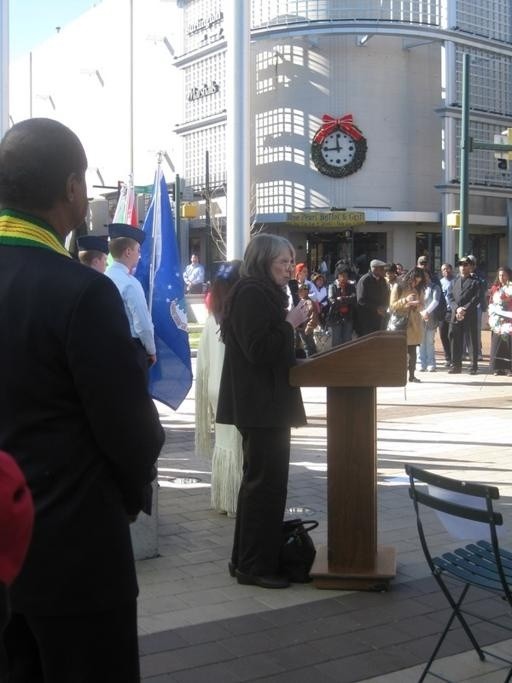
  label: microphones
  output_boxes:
[287,280,304,325]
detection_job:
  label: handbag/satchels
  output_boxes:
[278,518,319,583]
[388,311,409,331]
[431,283,447,321]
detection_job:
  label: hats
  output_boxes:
[458,257,471,264]
[371,259,387,267]
[311,272,325,282]
[418,256,431,264]
[77,235,109,255]
[108,223,147,245]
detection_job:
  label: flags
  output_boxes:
[131,168,194,411]
[107,185,139,267]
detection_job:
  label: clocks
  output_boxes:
[311,115,366,178]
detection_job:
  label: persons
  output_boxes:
[1,117,167,683]
[76,235,110,275]
[194,259,244,517]
[286,253,512,384]
[182,253,205,294]
[104,220,157,391]
[215,232,312,589]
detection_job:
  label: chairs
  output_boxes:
[403,462,512,683]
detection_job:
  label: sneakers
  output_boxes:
[229,562,290,588]
[408,353,512,382]
